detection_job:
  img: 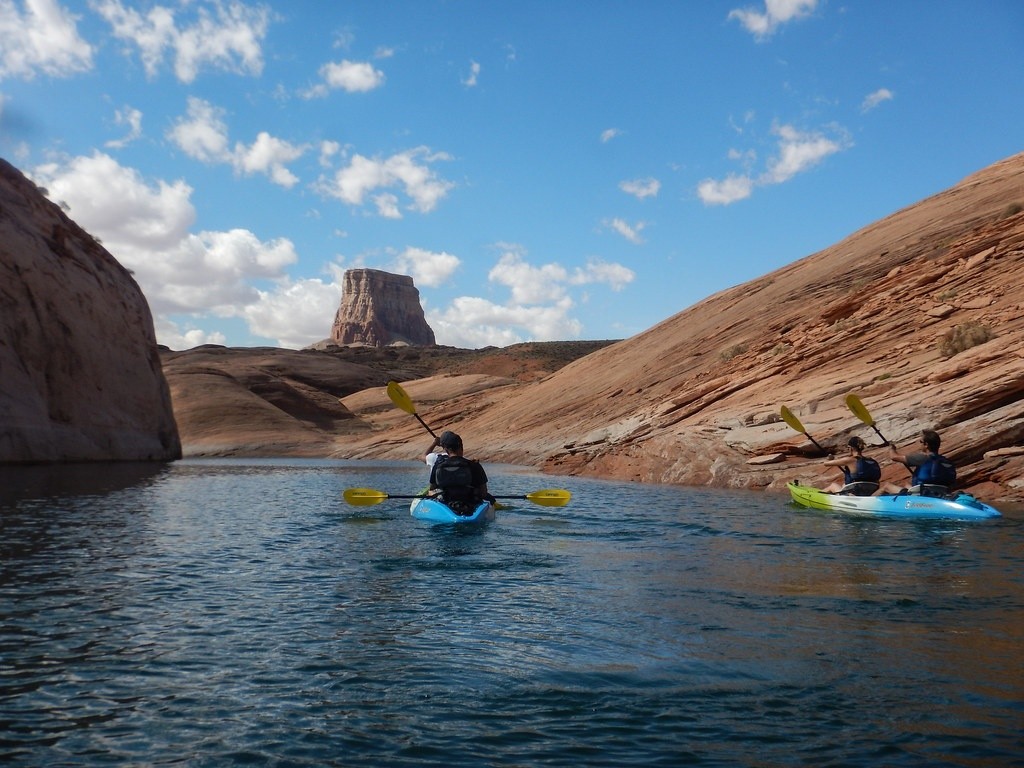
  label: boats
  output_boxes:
[785,480,1002,523]
[410,486,495,523]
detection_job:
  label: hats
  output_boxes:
[444,434,462,448]
[440,431,454,445]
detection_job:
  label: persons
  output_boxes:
[871,430,957,496]
[421,431,496,506]
[822,436,882,496]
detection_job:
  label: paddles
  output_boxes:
[385,380,504,510]
[778,403,846,474]
[341,488,572,508]
[845,393,915,475]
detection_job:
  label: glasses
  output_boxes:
[920,440,925,443]
